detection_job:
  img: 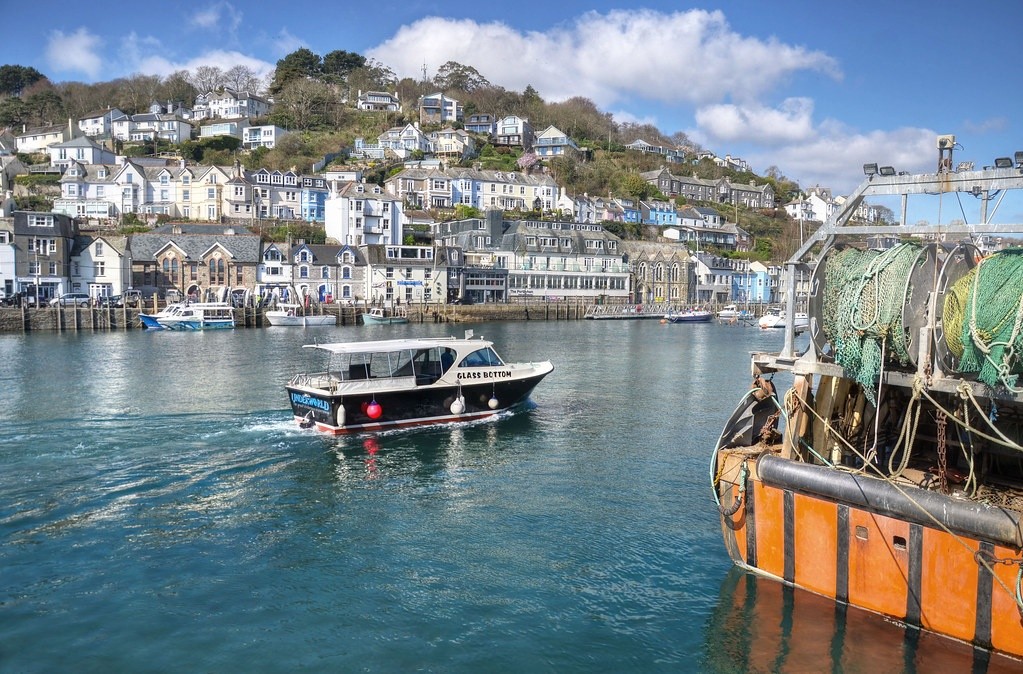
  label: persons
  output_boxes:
[441,347,454,376]
[97,293,102,308]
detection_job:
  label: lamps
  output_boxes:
[995,151,1023,169]
[863,164,895,177]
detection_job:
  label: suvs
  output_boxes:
[49,293,91,308]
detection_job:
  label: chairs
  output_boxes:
[348,363,378,380]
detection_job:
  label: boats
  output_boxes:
[264,302,337,326]
[137,300,237,331]
[717,257,754,321]
[286,329,551,439]
[362,306,410,325]
[708,134,1023,666]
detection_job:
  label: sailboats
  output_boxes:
[664,231,714,323]
[758,192,808,329]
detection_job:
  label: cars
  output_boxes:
[451,297,474,306]
[0,292,36,308]
[102,295,137,308]
